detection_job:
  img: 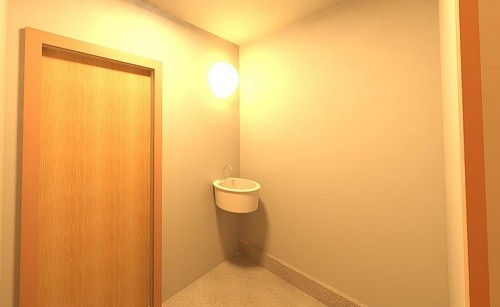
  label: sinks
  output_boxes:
[212,177,261,214]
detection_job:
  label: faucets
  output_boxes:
[223,164,233,180]
